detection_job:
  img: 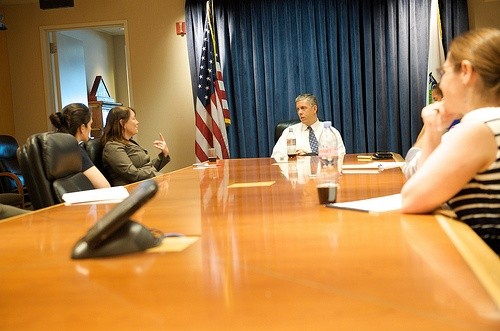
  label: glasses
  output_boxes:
[435,61,478,78]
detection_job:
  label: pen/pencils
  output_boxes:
[214,164,222,165]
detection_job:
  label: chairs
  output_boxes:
[0,172,24,209]
[0,135,34,203]
[275,119,301,145]
[17,129,94,212]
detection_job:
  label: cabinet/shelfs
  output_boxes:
[88,100,124,140]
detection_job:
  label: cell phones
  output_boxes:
[86,179,158,248]
[373,153,393,158]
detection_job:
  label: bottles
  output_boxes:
[316,120,338,205]
[286,127,297,159]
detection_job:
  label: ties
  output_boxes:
[307,126,318,152]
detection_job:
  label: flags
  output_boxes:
[195,0,231,163]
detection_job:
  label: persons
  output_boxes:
[271,93,346,158]
[49,103,110,189]
[100,106,171,187]
[400,26,500,259]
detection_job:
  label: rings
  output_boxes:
[161,144,163,146]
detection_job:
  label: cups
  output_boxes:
[207,148,218,163]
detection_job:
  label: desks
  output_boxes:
[0,152,500,331]
